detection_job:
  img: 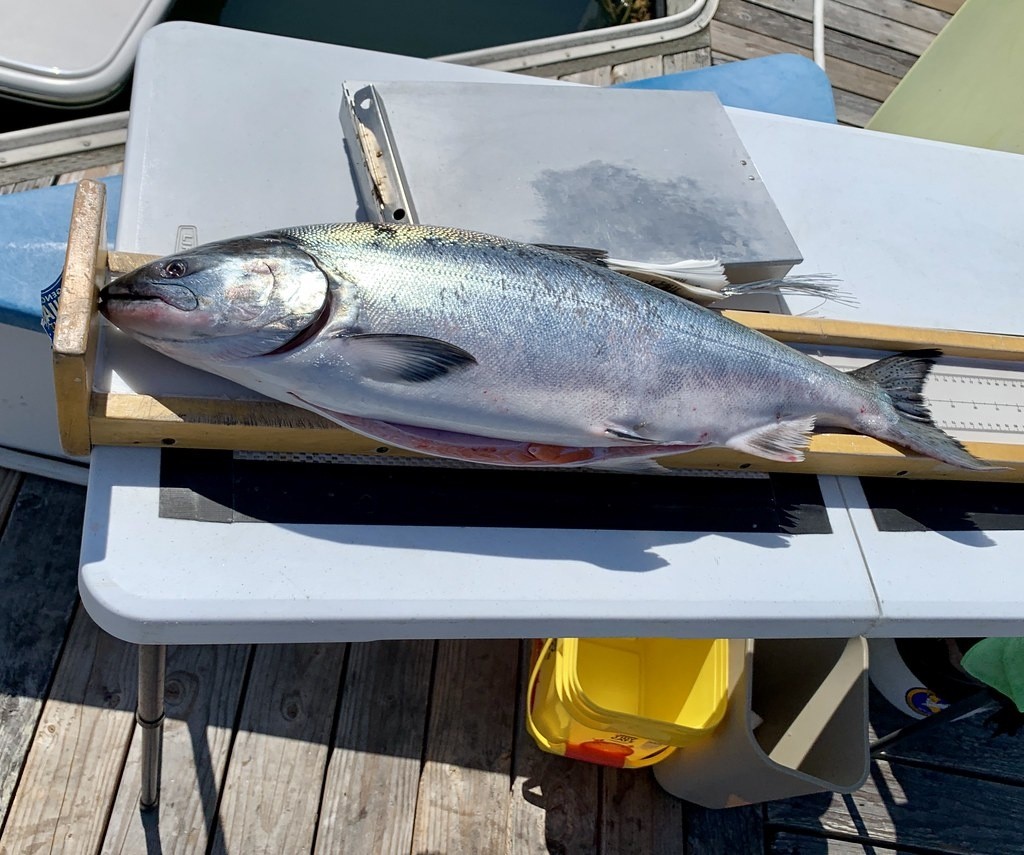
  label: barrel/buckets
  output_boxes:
[526,638,728,770]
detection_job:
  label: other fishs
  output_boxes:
[96,220,1016,477]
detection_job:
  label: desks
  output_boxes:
[76,22,1024,812]
